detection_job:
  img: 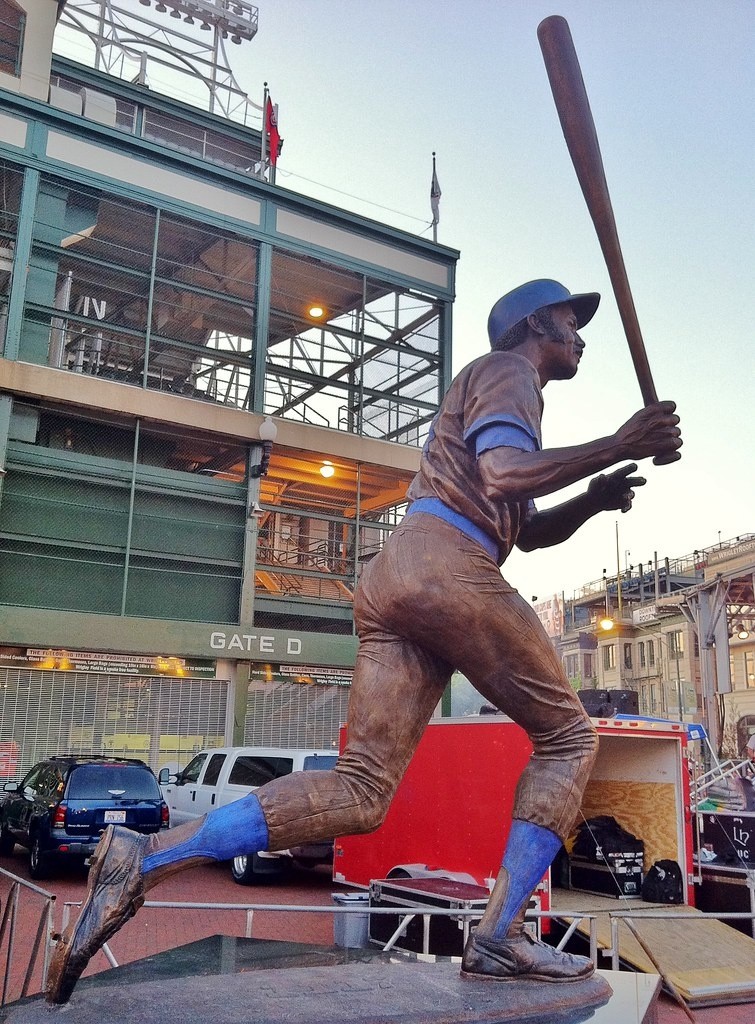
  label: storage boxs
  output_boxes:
[568,848,644,899]
[330,889,370,949]
[369,877,539,960]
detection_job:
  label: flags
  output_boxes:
[266,96,281,165]
[431,168,441,221]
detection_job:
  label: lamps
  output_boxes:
[249,417,279,478]
[735,624,749,640]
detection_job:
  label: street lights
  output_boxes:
[718,531,721,543]
[625,550,631,571]
[600,618,683,722]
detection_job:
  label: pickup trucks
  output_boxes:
[157,745,343,888]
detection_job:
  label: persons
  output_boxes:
[39,278,685,1005]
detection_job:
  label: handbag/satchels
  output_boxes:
[641,859,684,905]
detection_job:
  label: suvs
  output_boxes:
[0,752,172,880]
